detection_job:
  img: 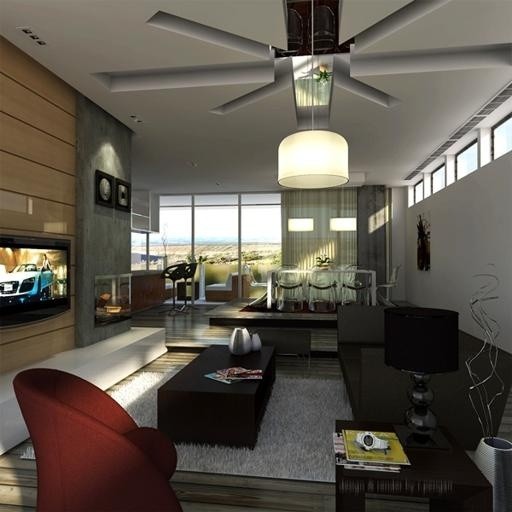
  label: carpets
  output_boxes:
[17,370,356,485]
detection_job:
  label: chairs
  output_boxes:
[160,262,190,317]
[242,262,402,311]
[12,366,183,512]
[179,262,201,313]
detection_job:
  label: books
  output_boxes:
[205,366,263,385]
[332,429,412,475]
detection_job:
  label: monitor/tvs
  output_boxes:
[0,234,70,326]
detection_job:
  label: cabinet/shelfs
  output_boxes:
[334,417,494,512]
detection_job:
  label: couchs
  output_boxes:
[335,304,512,451]
[205,271,238,301]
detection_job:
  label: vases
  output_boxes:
[472,437,512,512]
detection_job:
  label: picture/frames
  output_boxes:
[94,168,115,209]
[114,176,132,214]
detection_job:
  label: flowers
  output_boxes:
[311,252,338,270]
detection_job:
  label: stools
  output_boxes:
[249,329,312,370]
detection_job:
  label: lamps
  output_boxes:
[382,306,460,451]
[275,0,351,191]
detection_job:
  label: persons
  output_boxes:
[41,254,52,298]
